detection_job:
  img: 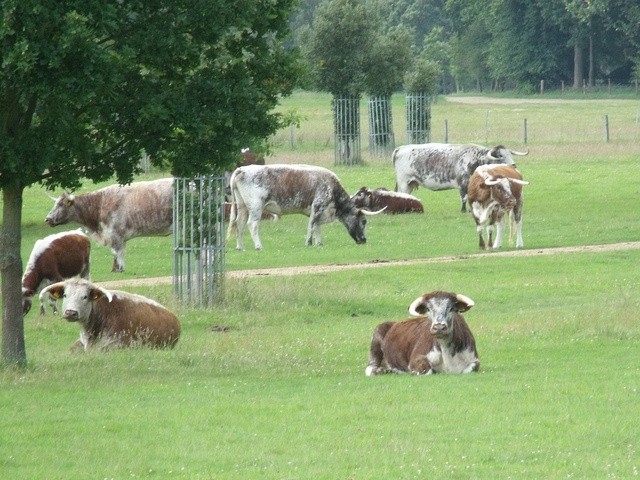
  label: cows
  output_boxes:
[350,187,426,217]
[21,227,93,318]
[364,291,482,377]
[38,276,183,353]
[220,203,277,224]
[467,163,532,250]
[391,141,531,213]
[225,163,390,252]
[44,175,222,274]
